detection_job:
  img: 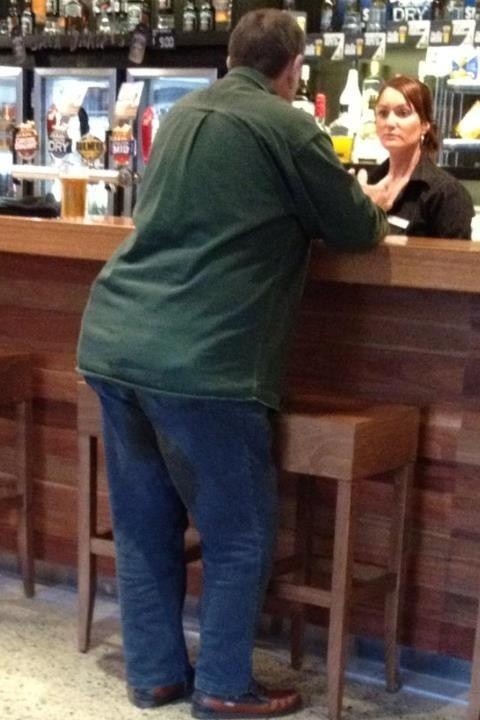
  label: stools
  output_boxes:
[0,347,38,601]
[265,394,421,719]
[74,378,204,655]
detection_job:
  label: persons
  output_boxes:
[75,5,395,719]
[361,75,476,240]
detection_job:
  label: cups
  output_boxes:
[57,178,92,220]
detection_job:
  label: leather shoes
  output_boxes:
[190,679,302,719]
[131,678,194,709]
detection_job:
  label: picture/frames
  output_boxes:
[0,63,220,213]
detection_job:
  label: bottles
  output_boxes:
[292,59,315,103]
[344,1,387,58]
[182,1,197,31]
[199,1,213,32]
[215,1,231,31]
[328,59,384,163]
[1,1,175,50]
